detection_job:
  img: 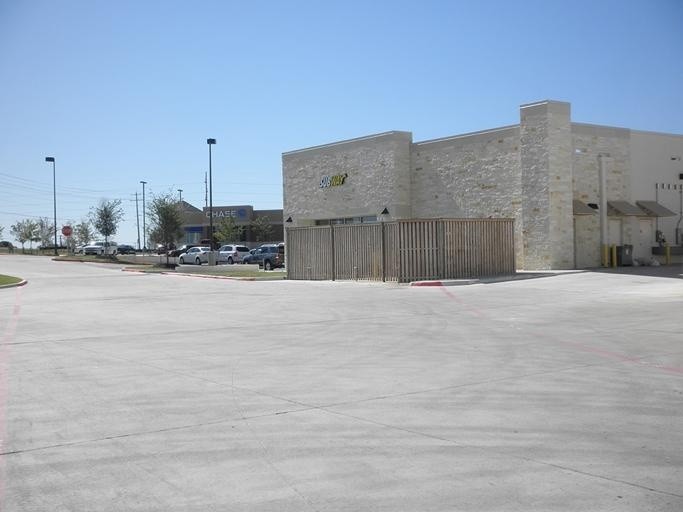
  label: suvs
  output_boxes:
[240,242,284,271]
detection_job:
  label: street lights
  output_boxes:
[139,180,147,254]
[206,136,217,266]
[44,155,61,256]
[177,188,183,202]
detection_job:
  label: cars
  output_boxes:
[217,243,251,264]
[73,239,199,258]
[178,246,219,265]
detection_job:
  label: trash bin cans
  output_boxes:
[616,244,634,266]
